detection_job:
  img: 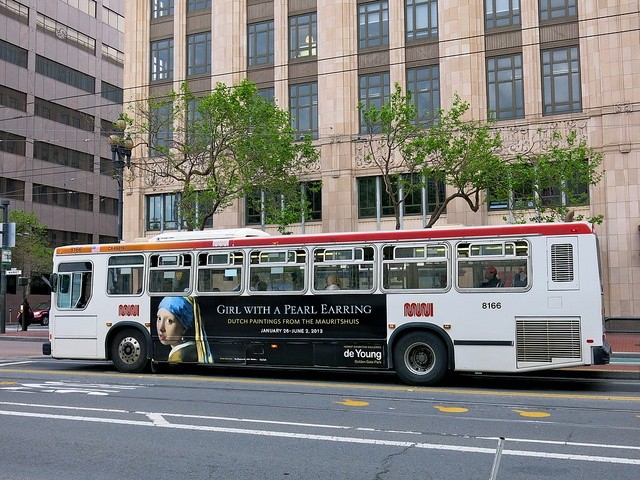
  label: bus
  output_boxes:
[44,219,613,386]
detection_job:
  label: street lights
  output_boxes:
[107,112,134,243]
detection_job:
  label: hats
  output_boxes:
[482,267,497,275]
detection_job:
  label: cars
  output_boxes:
[31,301,50,326]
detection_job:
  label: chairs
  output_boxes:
[496,280,505,287]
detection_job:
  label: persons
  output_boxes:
[251,275,259,291]
[258,281,267,291]
[514,262,527,287]
[478,266,500,287]
[325,275,342,290]
[157,296,198,362]
[432,271,447,288]
[19,298,30,331]
